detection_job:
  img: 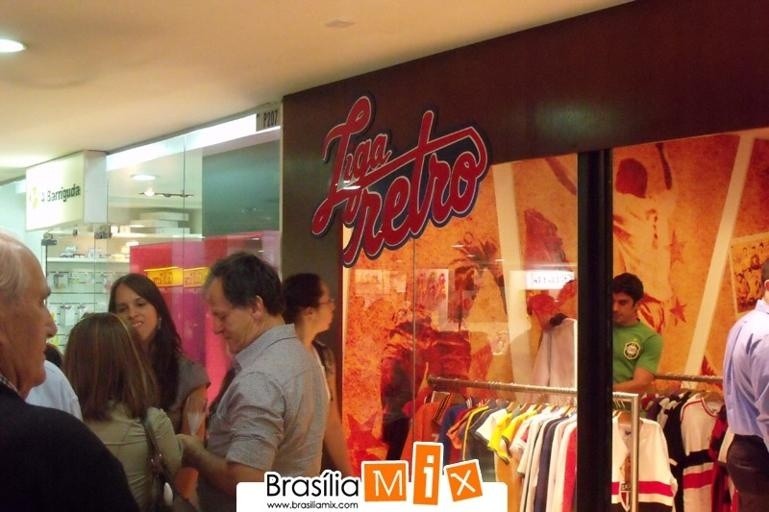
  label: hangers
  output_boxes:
[412,372,648,435]
[639,366,727,408]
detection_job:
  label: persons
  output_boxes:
[377,257,482,467]
[24,356,85,423]
[176,246,331,511]
[44,341,65,368]
[54,311,186,512]
[610,273,665,417]
[281,271,359,480]
[107,271,215,511]
[720,255,769,512]
[0,230,147,512]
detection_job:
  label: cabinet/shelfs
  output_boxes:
[39,223,204,361]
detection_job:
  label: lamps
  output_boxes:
[138,183,195,201]
[128,166,156,182]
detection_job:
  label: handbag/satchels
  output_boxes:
[140,414,199,512]
